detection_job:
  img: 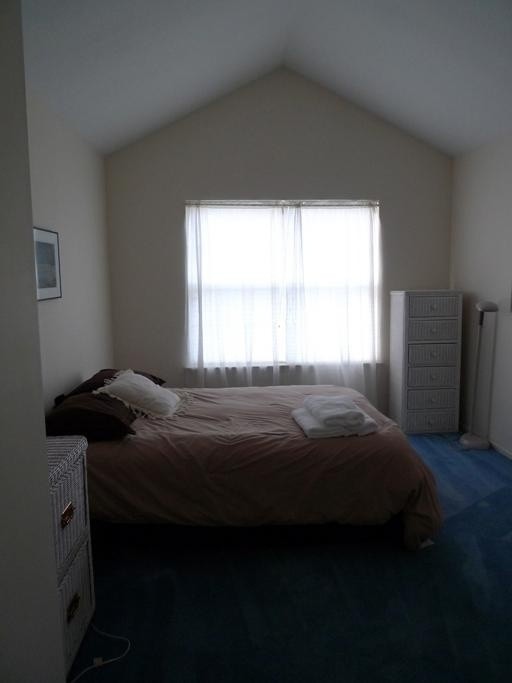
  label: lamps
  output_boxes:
[457,300,499,450]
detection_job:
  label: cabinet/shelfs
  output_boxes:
[387,288,463,435]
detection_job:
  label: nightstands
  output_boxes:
[46,434,98,671]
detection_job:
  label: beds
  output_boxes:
[52,385,428,542]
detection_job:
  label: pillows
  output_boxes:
[45,365,184,442]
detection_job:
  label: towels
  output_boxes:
[291,392,378,439]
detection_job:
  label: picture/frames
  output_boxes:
[32,224,63,301]
